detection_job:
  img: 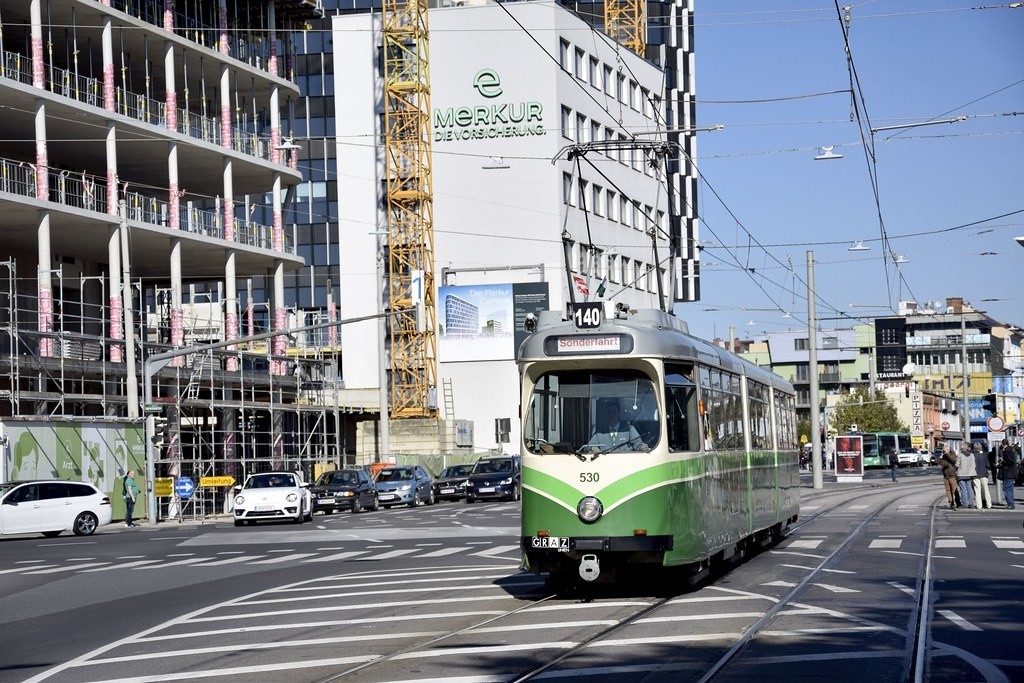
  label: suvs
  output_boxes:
[466,455,521,503]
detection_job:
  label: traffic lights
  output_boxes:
[905,386,910,397]
[951,389,955,399]
[983,394,996,412]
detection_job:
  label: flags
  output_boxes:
[597,277,607,297]
[573,276,589,294]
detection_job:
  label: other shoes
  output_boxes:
[893,480,899,482]
[1005,506,1015,510]
[959,505,974,509]
[941,506,953,509]
[952,505,958,508]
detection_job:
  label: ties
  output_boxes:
[611,428,617,441]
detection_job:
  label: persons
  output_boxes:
[122,471,140,527]
[800,450,812,471]
[889,450,900,482]
[583,404,649,452]
[406,470,413,478]
[939,439,1022,509]
[836,438,861,474]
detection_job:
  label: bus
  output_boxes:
[845,432,911,470]
[517,303,802,597]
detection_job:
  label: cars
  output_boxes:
[433,464,478,503]
[920,449,935,464]
[895,447,923,468]
[374,465,435,508]
[308,470,379,514]
[0,482,111,536]
[233,472,314,526]
